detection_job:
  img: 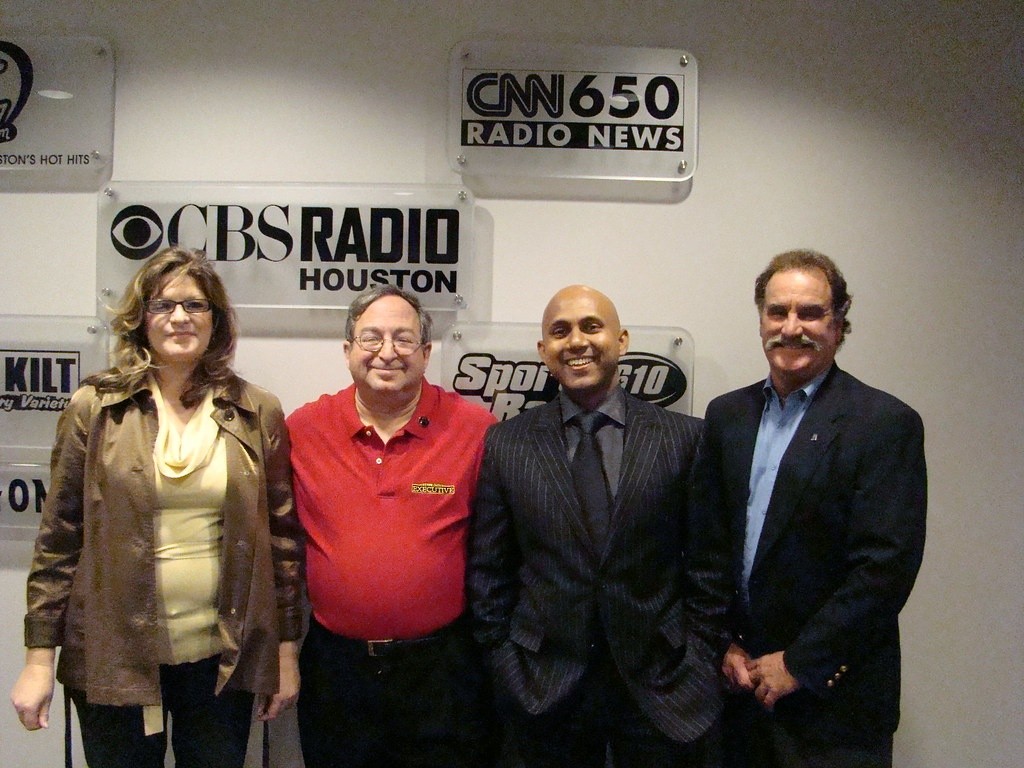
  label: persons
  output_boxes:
[284,283,500,768]
[463,285,733,768]
[10,245,305,768]
[703,248,928,768]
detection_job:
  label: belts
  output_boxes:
[343,638,443,657]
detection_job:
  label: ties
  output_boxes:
[568,410,614,560]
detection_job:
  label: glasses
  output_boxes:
[350,334,424,356]
[144,299,212,314]
